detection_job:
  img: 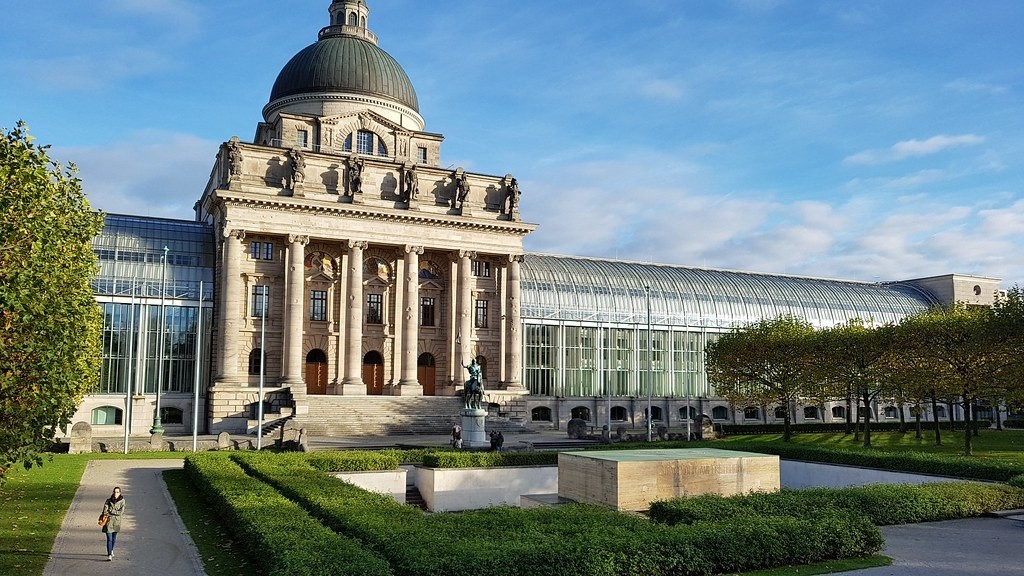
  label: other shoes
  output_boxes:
[108,555,112,561]
[111,550,114,557]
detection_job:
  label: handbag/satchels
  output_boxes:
[98,514,108,526]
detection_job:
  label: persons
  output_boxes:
[461,358,482,396]
[288,147,306,185]
[490,430,504,452]
[458,171,471,204]
[346,156,366,194]
[102,486,125,561]
[505,180,521,221]
[404,164,419,200]
[450,422,463,450]
[228,141,243,177]
